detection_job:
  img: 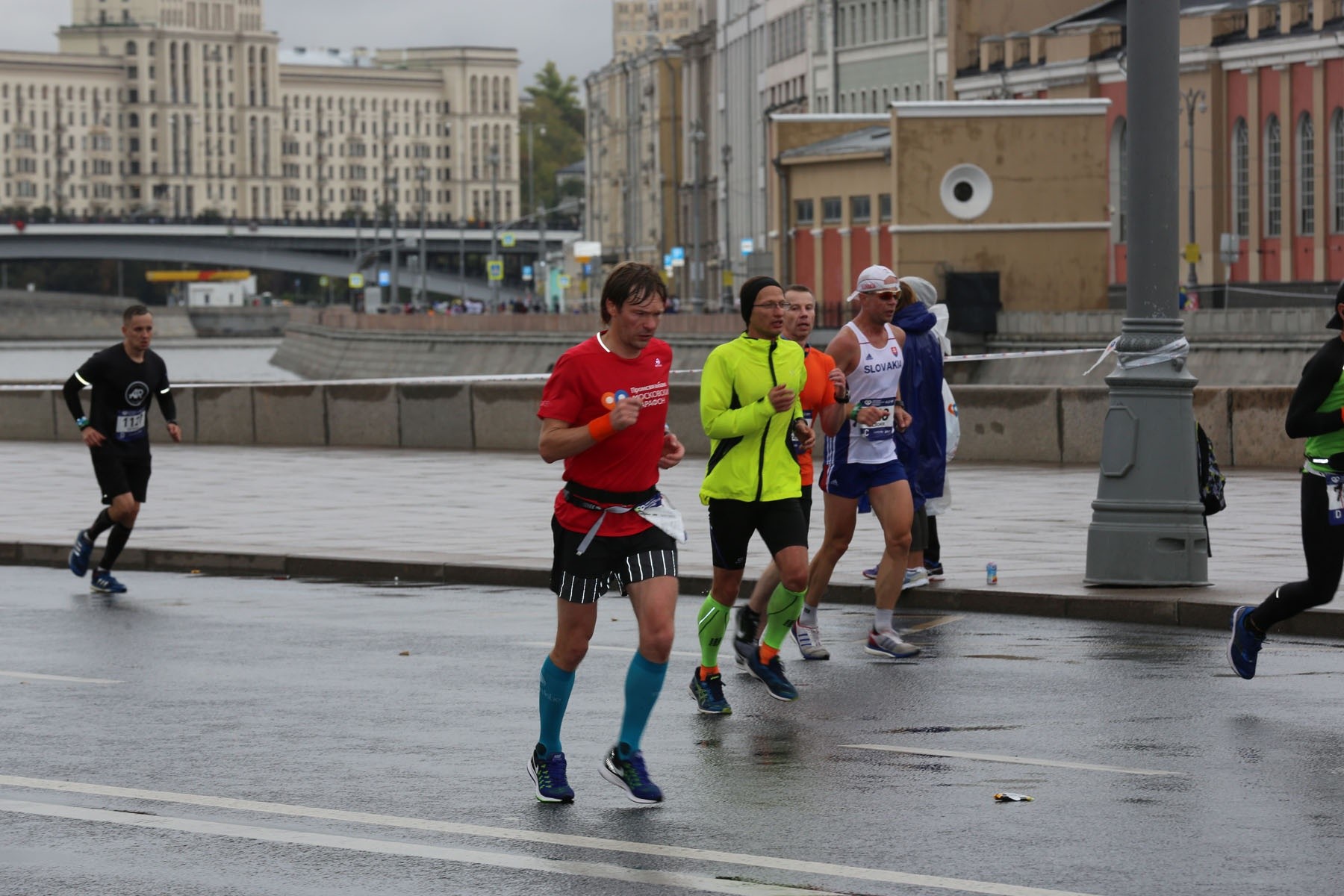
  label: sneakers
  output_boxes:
[597,744,663,804]
[527,743,575,802]
[90,566,127,593]
[734,652,746,670]
[789,618,830,660]
[745,646,798,702]
[733,605,760,659]
[864,626,921,658]
[923,562,946,581]
[1226,605,1269,680]
[68,527,94,578]
[687,666,733,714]
[862,563,879,578]
[900,569,930,590]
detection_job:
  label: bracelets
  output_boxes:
[589,412,616,442]
[664,424,670,436]
[850,405,861,428]
[792,418,808,429]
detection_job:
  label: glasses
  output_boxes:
[753,301,789,310]
[865,290,901,301]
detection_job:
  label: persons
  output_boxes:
[523,262,683,807]
[400,294,681,315]
[687,265,960,716]
[61,304,182,593]
[1229,283,1344,679]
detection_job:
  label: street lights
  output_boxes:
[1178,87,1210,289]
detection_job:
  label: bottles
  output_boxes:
[986,561,997,585]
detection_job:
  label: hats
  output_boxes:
[900,277,938,309]
[847,264,899,302]
[1325,280,1344,330]
[739,276,785,328]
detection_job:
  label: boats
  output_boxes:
[184,304,296,339]
[262,322,1123,385]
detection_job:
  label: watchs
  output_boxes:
[894,399,906,409]
